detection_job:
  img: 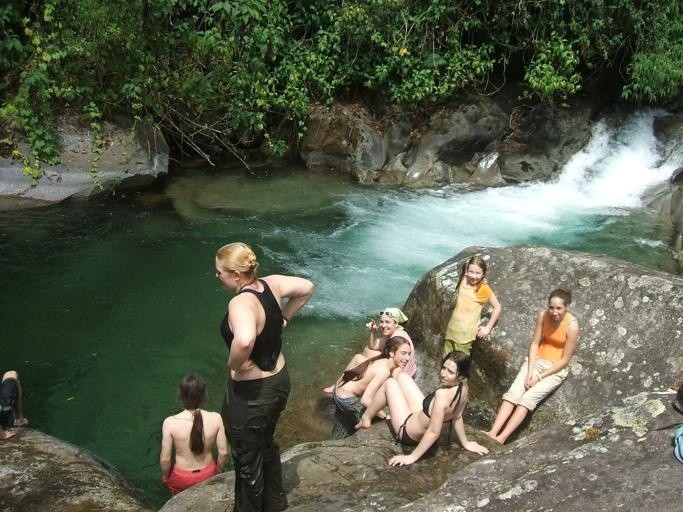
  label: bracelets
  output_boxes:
[384,412,388,419]
[282,314,290,323]
[537,374,542,381]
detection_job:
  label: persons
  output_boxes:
[1,370,29,440]
[354,350,490,467]
[442,256,502,360]
[330,335,411,439]
[479,289,577,445]
[160,374,228,495]
[323,308,416,394]
[215,242,313,511]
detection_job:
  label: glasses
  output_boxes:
[379,311,393,317]
